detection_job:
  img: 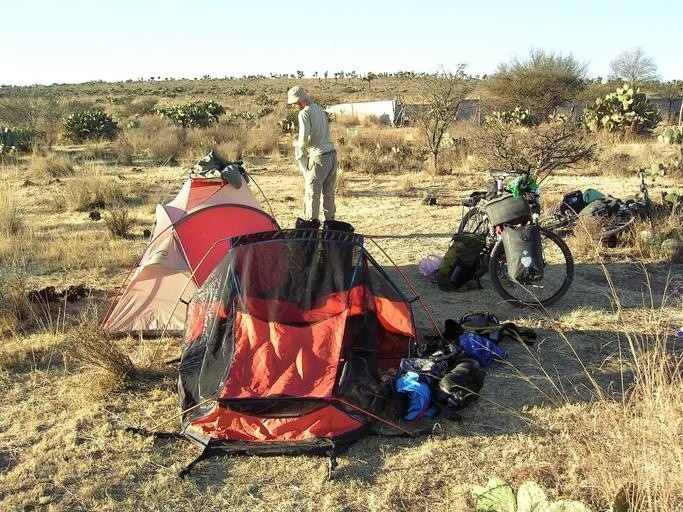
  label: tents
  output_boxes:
[149,220,451,457]
[96,149,292,342]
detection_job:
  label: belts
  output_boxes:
[322,150,337,155]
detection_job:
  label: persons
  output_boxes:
[286,86,337,220]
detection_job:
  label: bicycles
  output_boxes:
[457,164,573,309]
[538,168,656,246]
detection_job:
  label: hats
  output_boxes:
[287,85,309,105]
